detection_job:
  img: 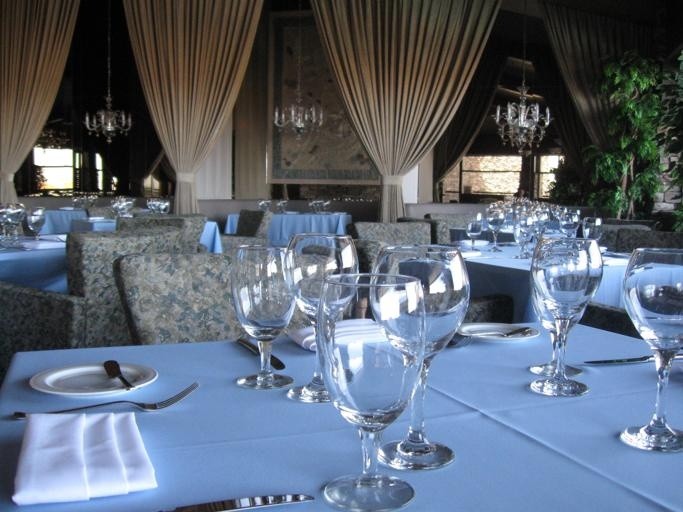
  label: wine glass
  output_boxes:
[314,271,418,512]
[226,242,301,388]
[617,246,683,451]
[284,234,362,403]
[469,192,603,264]
[367,239,472,473]
[256,198,332,217]
[529,236,603,397]
[0,194,173,251]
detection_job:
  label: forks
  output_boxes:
[466,325,530,337]
[13,379,201,422]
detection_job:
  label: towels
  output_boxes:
[11,407,157,505]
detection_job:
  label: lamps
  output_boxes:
[82,13,135,147]
[31,121,69,153]
[271,54,327,139]
[495,49,554,158]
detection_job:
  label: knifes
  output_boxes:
[103,357,138,392]
[237,338,285,371]
[582,352,683,365]
[169,492,314,512]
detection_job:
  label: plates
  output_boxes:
[26,365,157,397]
[458,322,539,344]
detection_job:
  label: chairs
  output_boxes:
[0,187,683,348]
